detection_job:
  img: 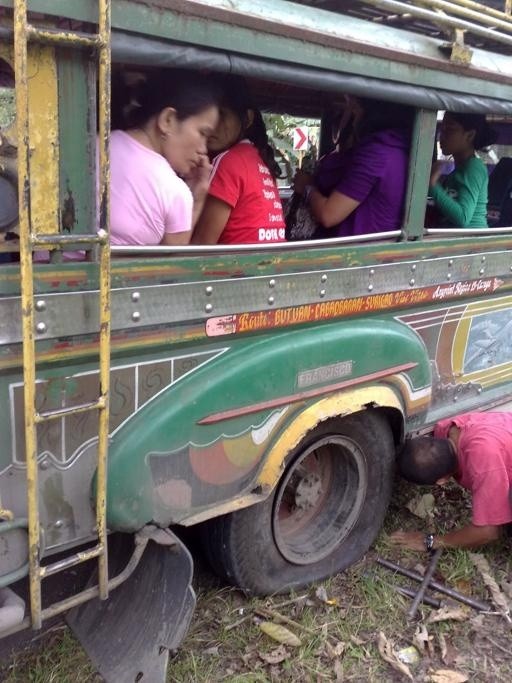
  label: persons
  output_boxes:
[106,67,224,247]
[290,91,407,241]
[425,108,498,230]
[191,73,289,245]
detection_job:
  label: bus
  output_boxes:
[0,2,512,682]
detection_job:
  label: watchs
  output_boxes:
[425,534,434,551]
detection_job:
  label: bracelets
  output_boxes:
[302,182,318,205]
[385,411,512,556]
